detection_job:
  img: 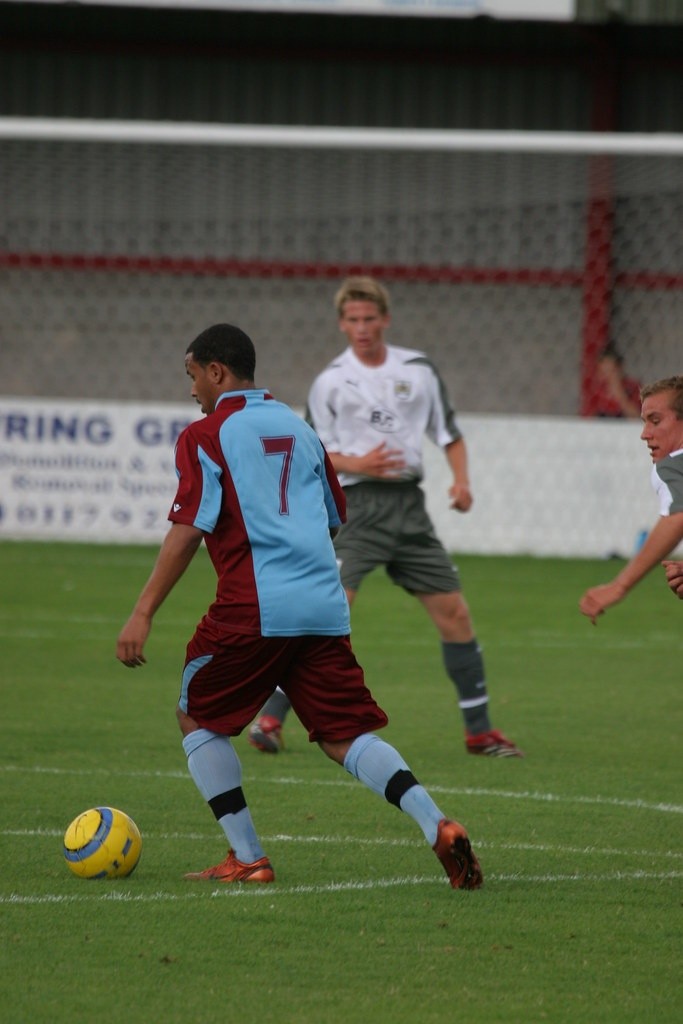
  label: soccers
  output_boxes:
[62,805,143,881]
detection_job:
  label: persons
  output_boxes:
[116,324,482,888]
[248,274,519,757]
[580,376,683,626]
[579,342,641,419]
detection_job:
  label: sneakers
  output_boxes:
[183,848,275,882]
[435,819,483,889]
[465,727,522,758]
[249,717,281,751]
